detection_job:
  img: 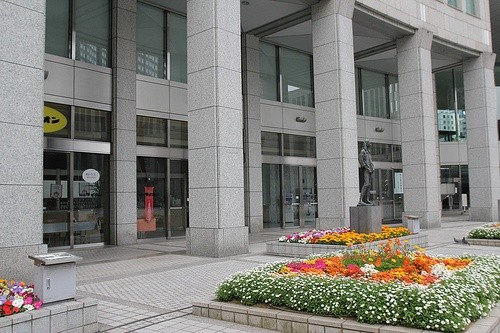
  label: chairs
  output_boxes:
[42,208,101,247]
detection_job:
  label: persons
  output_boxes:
[357,140,375,206]
